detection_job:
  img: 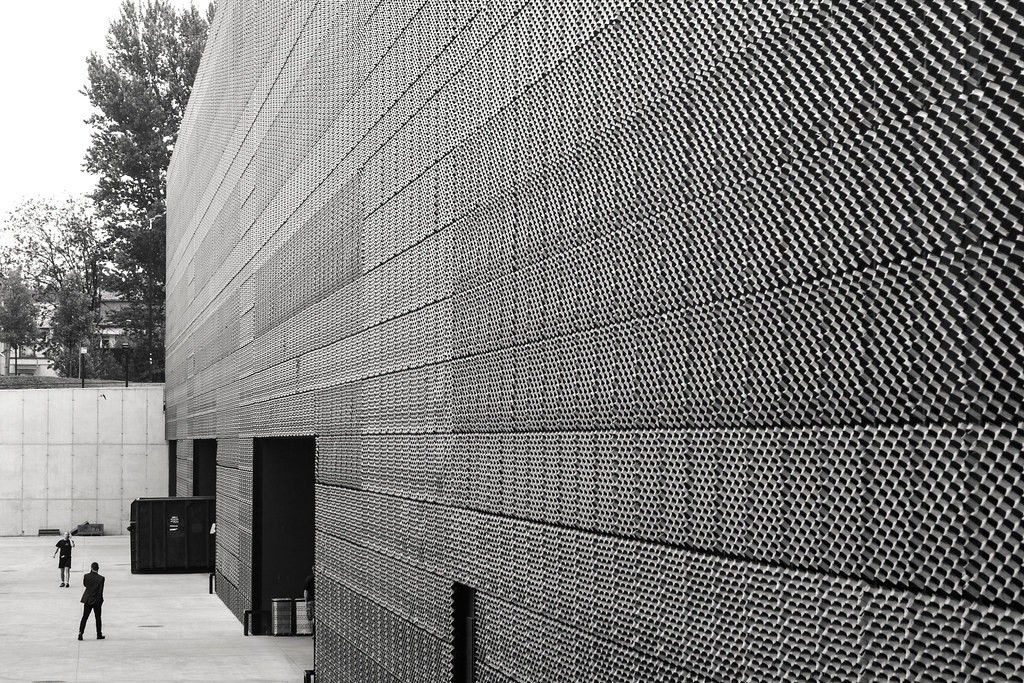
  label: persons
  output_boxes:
[78,563,106,641]
[53,531,75,588]
[304,566,315,639]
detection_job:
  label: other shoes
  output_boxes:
[97,636,105,639]
[60,583,65,587]
[78,634,83,640]
[66,583,69,587]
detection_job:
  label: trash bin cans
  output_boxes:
[272,598,291,636]
[295,599,314,635]
